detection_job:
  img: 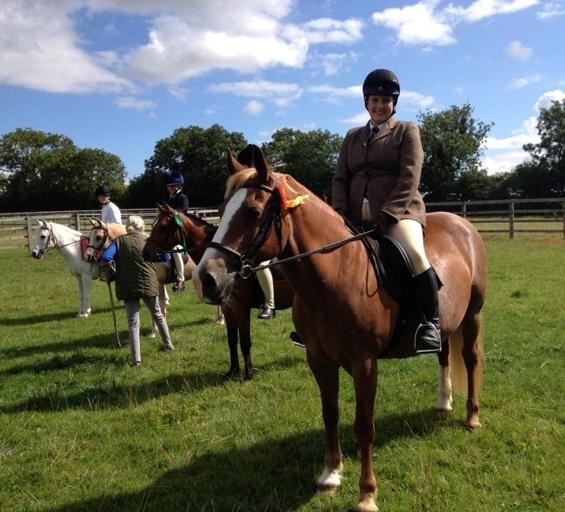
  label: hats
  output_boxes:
[366,81,399,95]
[95,184,110,196]
[165,170,184,186]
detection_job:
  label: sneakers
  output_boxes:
[131,360,141,367]
[165,345,174,352]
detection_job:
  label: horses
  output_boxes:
[85,214,195,338]
[190,143,489,512]
[31,218,117,318]
[140,198,292,383]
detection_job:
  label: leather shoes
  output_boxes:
[258,308,276,318]
[173,280,185,290]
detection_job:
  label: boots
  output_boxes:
[413,267,442,349]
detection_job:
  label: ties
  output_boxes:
[367,127,379,143]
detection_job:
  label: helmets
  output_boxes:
[363,69,400,100]
[237,144,253,165]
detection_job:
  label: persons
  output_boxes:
[102,215,175,369]
[95,185,122,282]
[159,170,189,292]
[235,144,279,320]
[288,69,442,354]
